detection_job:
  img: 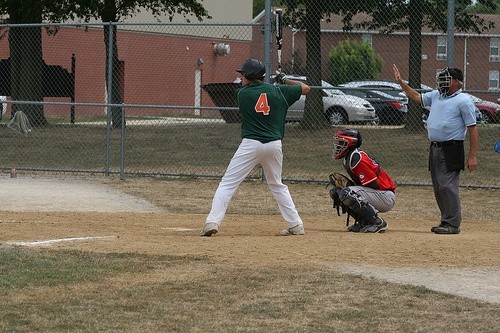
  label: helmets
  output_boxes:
[332,128,362,160]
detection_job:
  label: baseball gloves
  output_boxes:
[326,173,355,190]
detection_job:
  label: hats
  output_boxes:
[439,66,464,80]
[235,59,267,80]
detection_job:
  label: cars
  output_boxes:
[333,86,408,124]
[348,78,436,121]
[464,92,500,124]
[233,74,376,126]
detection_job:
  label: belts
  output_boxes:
[432,141,458,147]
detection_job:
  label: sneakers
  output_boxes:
[279,225,305,235]
[347,217,388,232]
[201,223,218,236]
[432,223,460,234]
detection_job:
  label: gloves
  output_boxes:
[270,70,286,84]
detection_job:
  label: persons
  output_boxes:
[329,130,396,232]
[392,63,478,234]
[199,59,310,236]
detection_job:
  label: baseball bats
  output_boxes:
[275,10,283,73]
